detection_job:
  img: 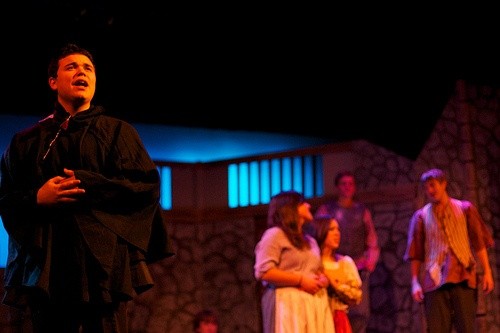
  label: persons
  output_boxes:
[255,191,335,333]
[308,215,363,333]
[0,48,161,333]
[314,173,380,333]
[192,309,221,333]
[403,169,494,333]
